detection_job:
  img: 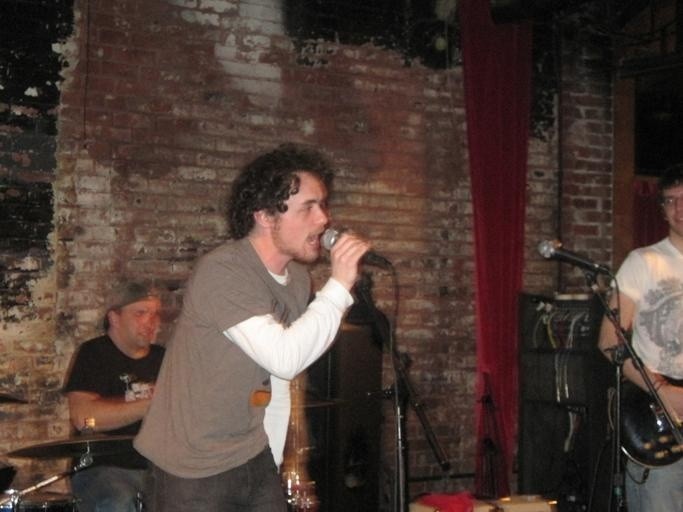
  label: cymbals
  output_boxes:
[4,419,143,458]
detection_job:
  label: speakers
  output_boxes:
[308,325,380,509]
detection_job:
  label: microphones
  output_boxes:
[537,241,607,276]
[321,230,390,274]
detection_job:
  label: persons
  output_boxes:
[58,276,165,511]
[598,161,683,512]
[134,144,373,512]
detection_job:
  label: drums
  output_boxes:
[0,492,72,510]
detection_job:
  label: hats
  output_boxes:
[104,282,158,331]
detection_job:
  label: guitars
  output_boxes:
[608,371,683,469]
